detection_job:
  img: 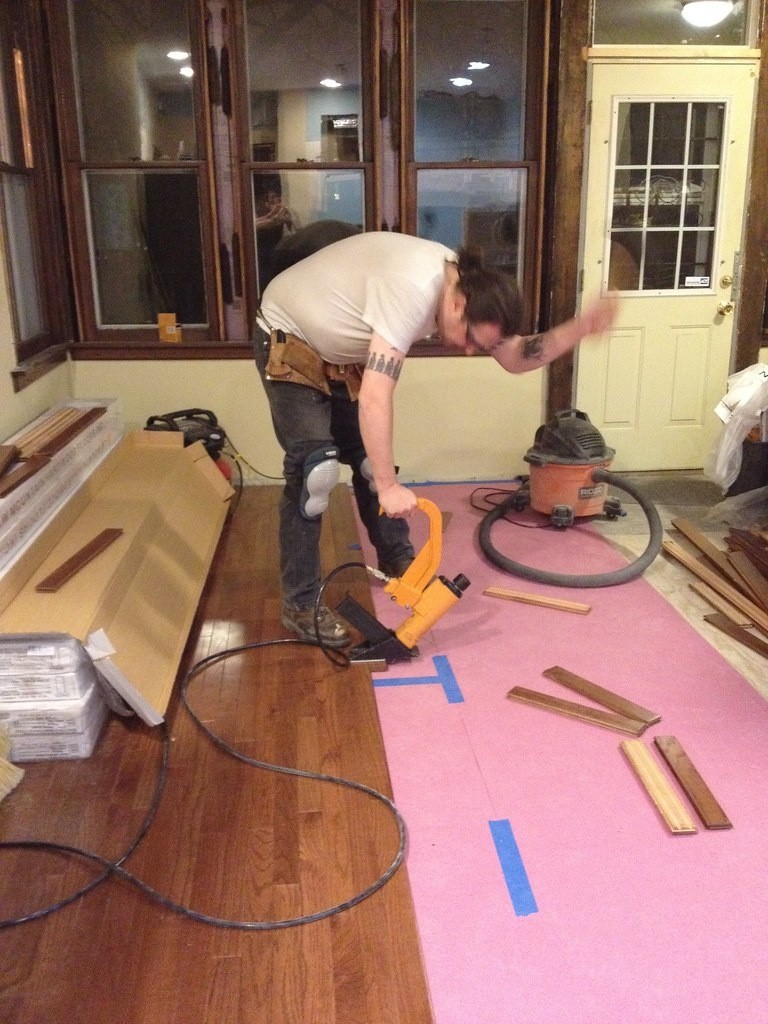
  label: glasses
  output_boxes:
[465,320,496,356]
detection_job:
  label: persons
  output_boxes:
[252,230,620,646]
[254,181,291,256]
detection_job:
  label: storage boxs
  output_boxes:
[0,396,235,760]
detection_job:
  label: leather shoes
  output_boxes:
[281,603,352,647]
[378,556,437,588]
